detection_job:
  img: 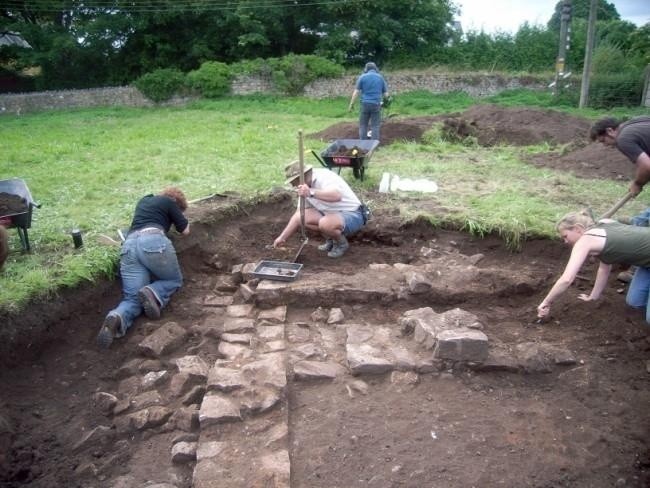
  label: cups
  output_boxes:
[72,228,84,247]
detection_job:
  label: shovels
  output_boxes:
[186,192,228,204]
[293,130,308,263]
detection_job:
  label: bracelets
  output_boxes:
[350,103,354,105]
[310,191,314,198]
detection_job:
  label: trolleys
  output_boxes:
[311,138,381,179]
[0,177,43,254]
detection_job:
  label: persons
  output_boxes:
[348,62,389,139]
[273,159,366,259]
[95,187,191,350]
[536,210,650,326]
[586,115,650,198]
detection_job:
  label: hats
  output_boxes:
[285,160,313,184]
[364,63,380,73]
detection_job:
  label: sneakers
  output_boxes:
[319,238,349,258]
[618,267,636,281]
[139,287,160,319]
[97,314,120,350]
